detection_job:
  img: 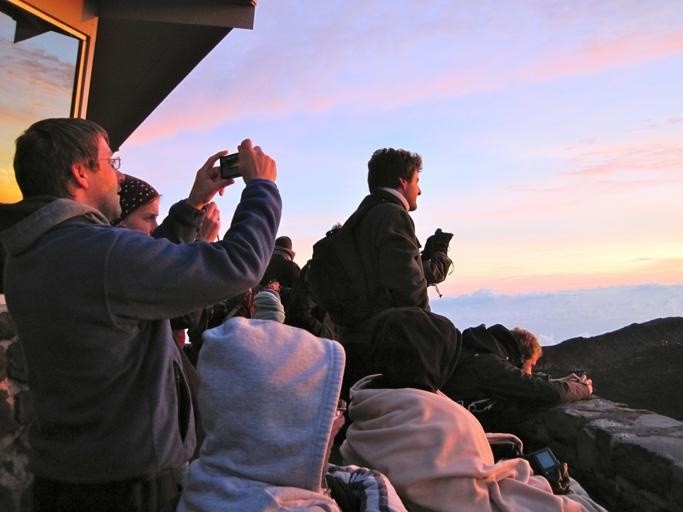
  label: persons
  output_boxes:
[192,317,609,512]
[304,146,452,399]
[0,117,301,512]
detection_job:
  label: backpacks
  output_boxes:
[307,194,401,329]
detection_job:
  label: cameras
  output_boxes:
[220,153,243,178]
[526,448,569,495]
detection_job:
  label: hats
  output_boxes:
[370,306,465,390]
[110,173,159,226]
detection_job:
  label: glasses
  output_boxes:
[95,155,122,169]
